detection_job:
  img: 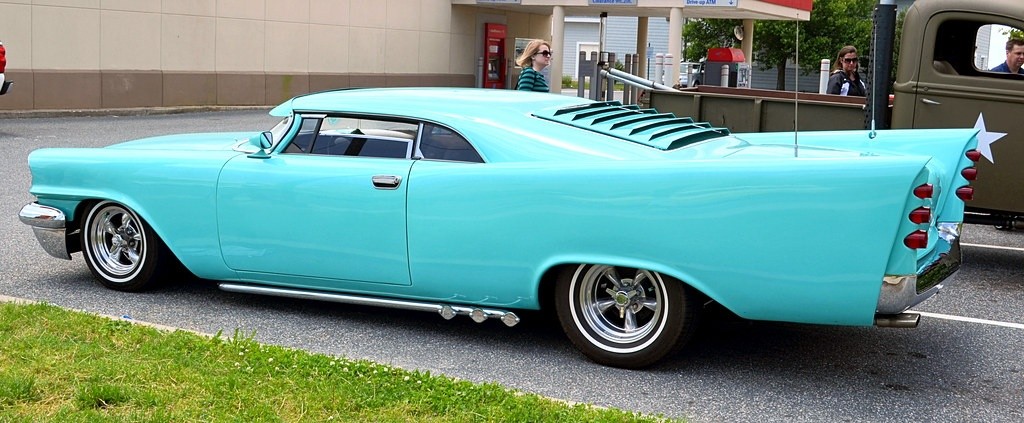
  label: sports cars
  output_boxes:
[15,86,984,370]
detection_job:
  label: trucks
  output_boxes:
[637,1,1024,237]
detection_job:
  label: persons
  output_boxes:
[989,37,1024,77]
[517,38,552,93]
[826,46,866,97]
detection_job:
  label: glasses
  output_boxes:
[842,58,858,63]
[534,50,551,57]
